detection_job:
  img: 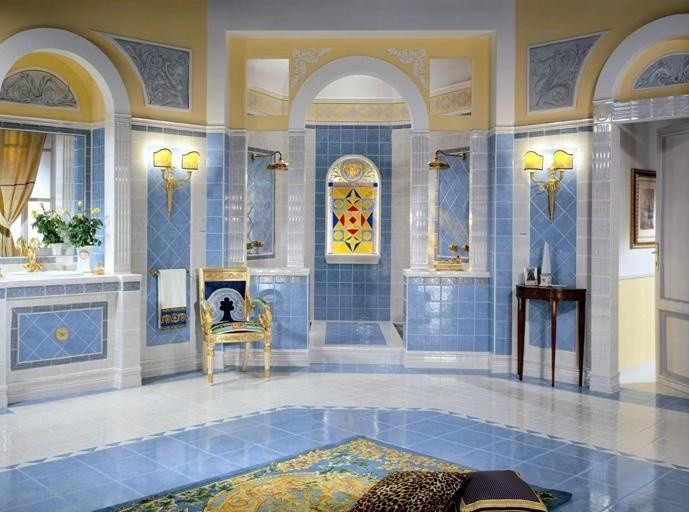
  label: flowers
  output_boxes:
[61,199,104,247]
[29,202,69,247]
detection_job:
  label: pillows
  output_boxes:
[342,469,548,512]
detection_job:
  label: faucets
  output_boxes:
[24,237,44,271]
[16,235,28,255]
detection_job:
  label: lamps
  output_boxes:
[151,145,201,217]
[426,148,467,172]
[251,149,290,171]
[522,148,575,224]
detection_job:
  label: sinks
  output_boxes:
[7,269,84,279]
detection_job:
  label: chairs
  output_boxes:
[198,266,274,386]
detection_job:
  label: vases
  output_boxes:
[75,243,94,274]
[49,242,65,256]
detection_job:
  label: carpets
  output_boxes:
[91,435,572,511]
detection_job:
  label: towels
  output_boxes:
[155,268,190,330]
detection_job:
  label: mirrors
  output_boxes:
[1,121,92,263]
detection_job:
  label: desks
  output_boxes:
[515,283,586,387]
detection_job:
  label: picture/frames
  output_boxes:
[629,167,657,248]
